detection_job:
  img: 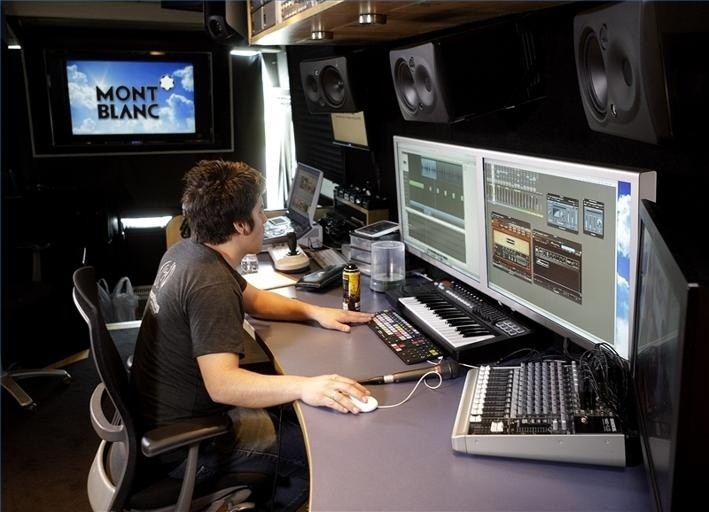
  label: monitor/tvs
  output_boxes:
[327,111,371,153]
[286,162,324,225]
[392,136,484,294]
[477,148,656,371]
[43,51,215,148]
[629,198,709,511]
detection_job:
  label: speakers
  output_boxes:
[388,5,570,123]
[298,46,400,115]
[572,1,709,145]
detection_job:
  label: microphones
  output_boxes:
[358,358,460,385]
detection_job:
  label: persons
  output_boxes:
[126,154,374,511]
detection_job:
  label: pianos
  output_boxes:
[385,276,534,361]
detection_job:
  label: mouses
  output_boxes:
[346,395,378,414]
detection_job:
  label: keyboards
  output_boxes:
[366,308,450,364]
[310,247,347,270]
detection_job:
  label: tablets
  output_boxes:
[354,220,398,238]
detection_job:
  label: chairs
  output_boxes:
[69,266,252,511]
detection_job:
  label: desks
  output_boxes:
[240,205,657,512]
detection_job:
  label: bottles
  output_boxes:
[341,264,361,312]
